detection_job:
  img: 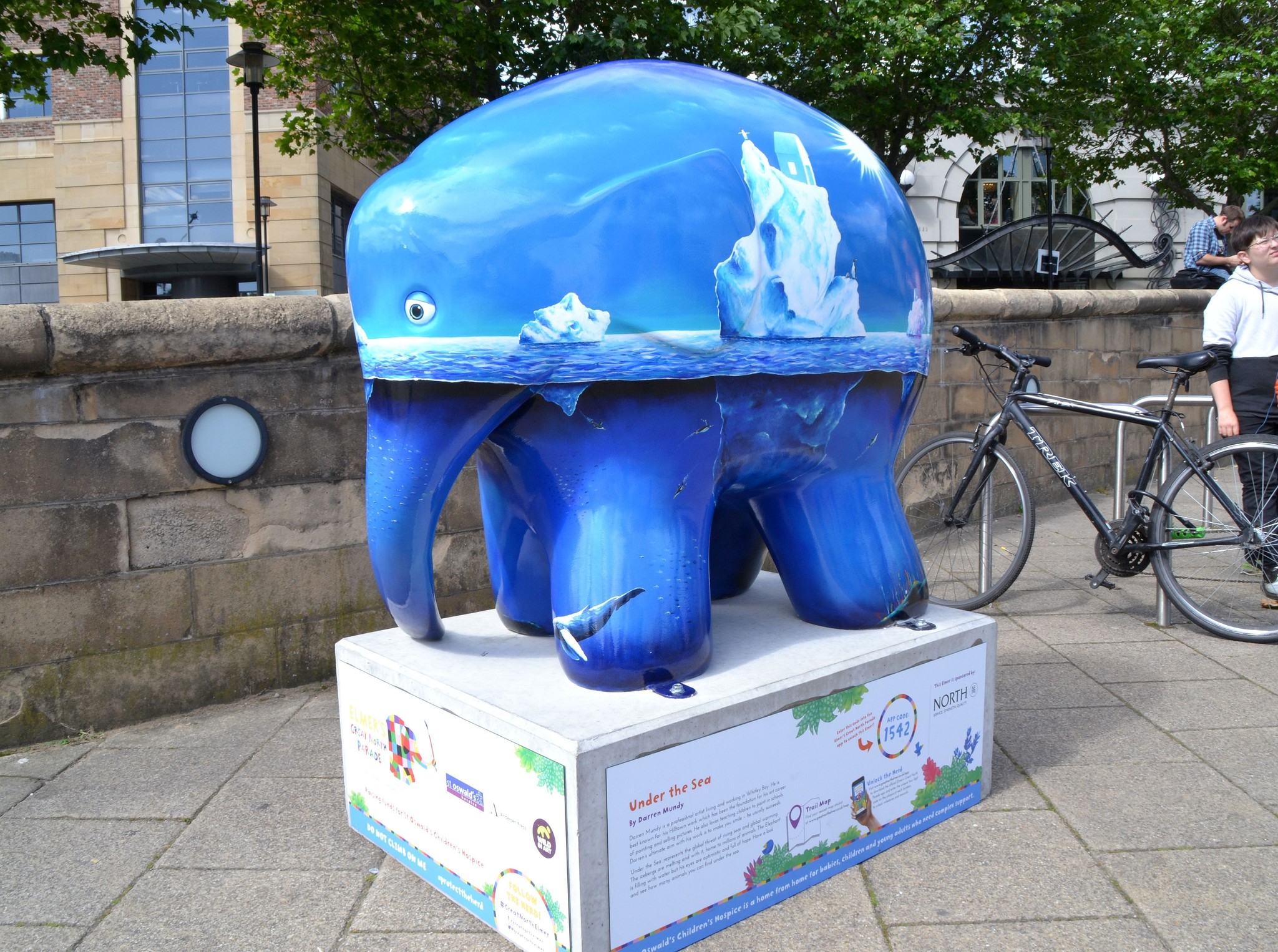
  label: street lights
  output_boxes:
[1041,119,1057,290]
[226,42,281,295]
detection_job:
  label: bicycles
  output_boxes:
[895,324,1278,642]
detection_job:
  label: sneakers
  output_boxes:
[1260,564,1278,600]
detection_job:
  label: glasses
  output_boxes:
[1227,218,1234,229]
[1244,231,1278,251]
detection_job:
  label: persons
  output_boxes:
[1202,213,1278,602]
[1183,205,1245,278]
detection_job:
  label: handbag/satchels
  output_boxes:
[1170,268,1226,290]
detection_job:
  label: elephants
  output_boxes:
[346,58,932,694]
